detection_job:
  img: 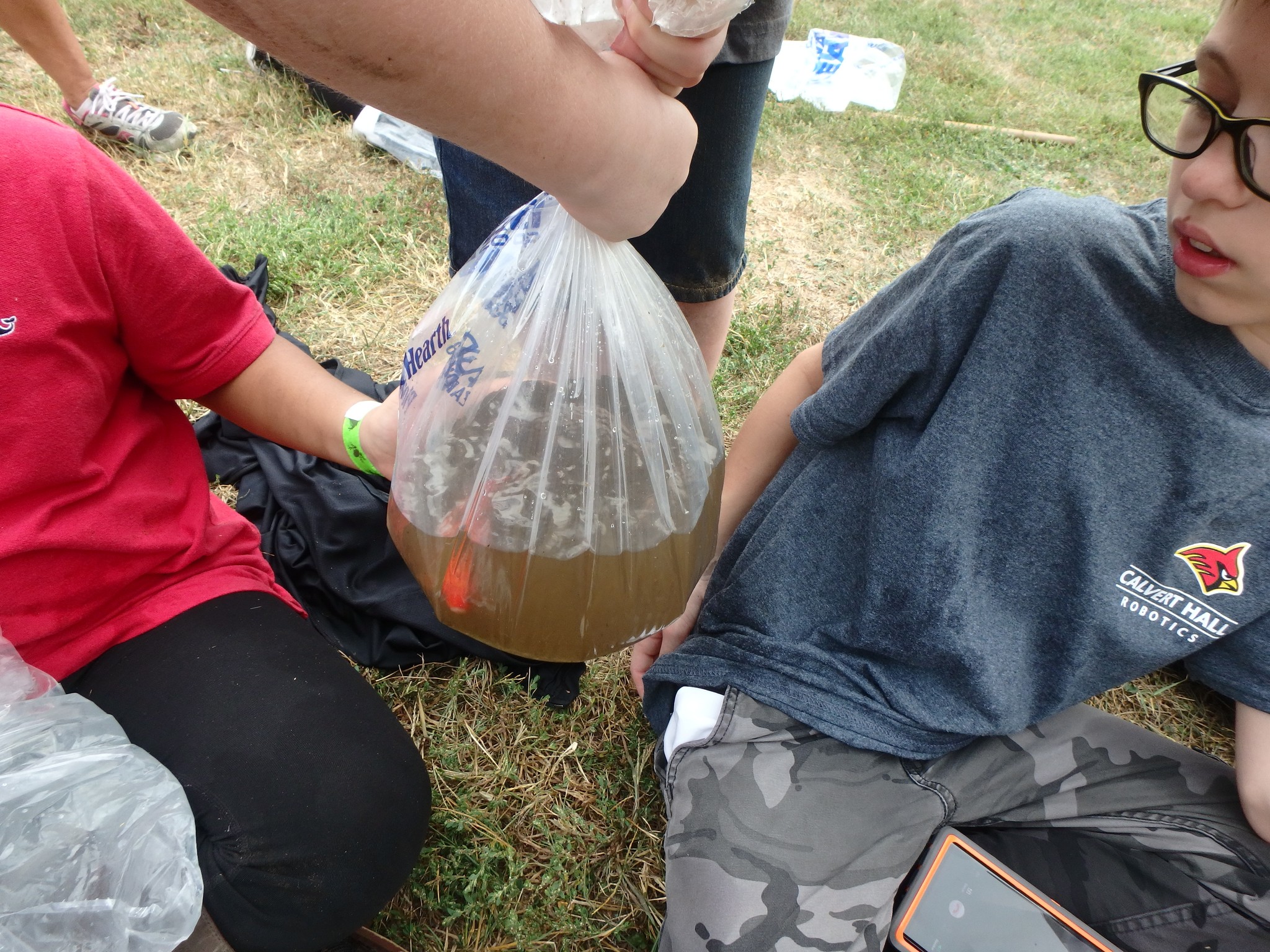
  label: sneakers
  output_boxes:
[63,76,198,155]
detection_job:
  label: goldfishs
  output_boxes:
[440,479,513,614]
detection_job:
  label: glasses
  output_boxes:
[1137,60,1270,202]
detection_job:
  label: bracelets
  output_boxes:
[343,400,384,477]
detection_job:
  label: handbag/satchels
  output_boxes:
[385,1,758,665]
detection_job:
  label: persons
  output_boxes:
[0,101,453,952]
[624,2,1270,952]
[433,0,792,382]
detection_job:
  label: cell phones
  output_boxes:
[884,825,1124,952]
